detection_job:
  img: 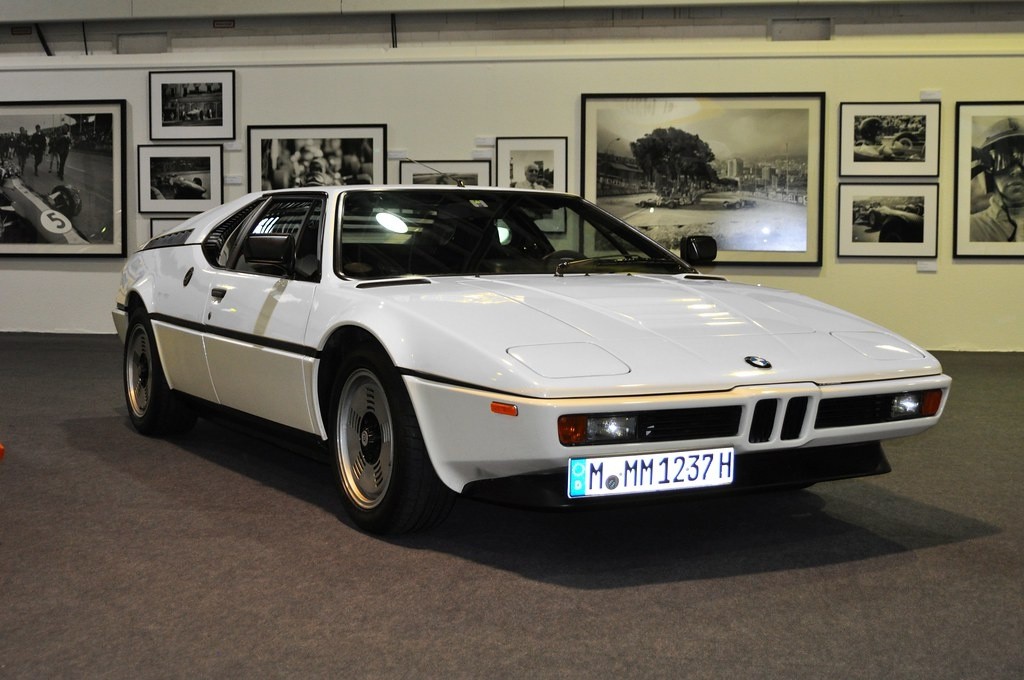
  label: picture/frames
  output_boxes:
[399,158,492,189]
[496,136,569,233]
[150,217,190,240]
[839,101,940,177]
[837,182,939,257]
[246,124,389,193]
[148,70,237,140]
[953,100,1024,258]
[0,99,127,258]
[580,92,825,267]
[137,143,224,214]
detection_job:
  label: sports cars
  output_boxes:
[111,156,953,538]
[723,199,757,210]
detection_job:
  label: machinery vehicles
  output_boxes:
[852,199,924,244]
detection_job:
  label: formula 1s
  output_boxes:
[0,158,91,246]
